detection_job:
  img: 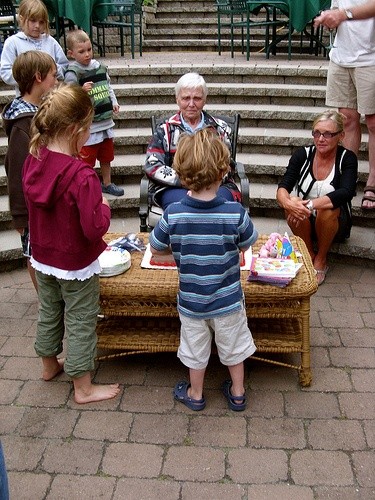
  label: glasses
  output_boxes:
[311,130,343,139]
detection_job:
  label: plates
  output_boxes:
[98,247,131,277]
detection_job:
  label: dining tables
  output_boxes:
[16,0,116,58]
[246,0,332,61]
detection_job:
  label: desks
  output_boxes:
[95,231,318,388]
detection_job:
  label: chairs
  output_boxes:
[213,0,335,61]
[0,0,143,60]
[140,113,250,233]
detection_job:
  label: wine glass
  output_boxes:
[321,10,337,49]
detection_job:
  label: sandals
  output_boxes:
[221,379,248,411]
[101,183,124,196]
[172,381,206,411]
[360,185,375,211]
[317,267,329,285]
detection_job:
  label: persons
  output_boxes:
[276,111,358,285]
[149,127,259,412]
[0,0,69,100]
[64,30,125,196]
[313,0,375,209]
[144,72,241,211]
[1,50,59,295]
[22,86,121,404]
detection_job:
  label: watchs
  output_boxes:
[344,7,354,20]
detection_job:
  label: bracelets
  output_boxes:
[305,200,313,211]
